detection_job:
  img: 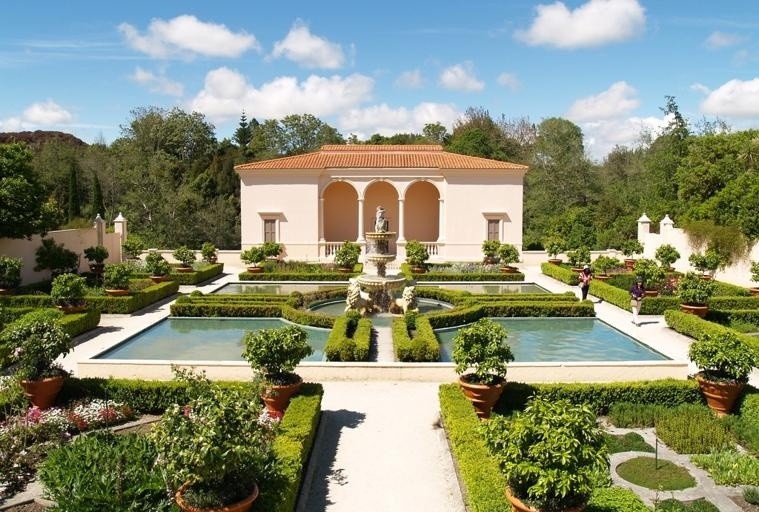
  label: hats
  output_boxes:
[582,265,589,271]
[635,278,643,283]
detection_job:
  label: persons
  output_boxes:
[577,264,594,302]
[629,277,648,327]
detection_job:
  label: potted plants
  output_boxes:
[546,236,759,321]
[240,246,265,273]
[410,244,430,273]
[451,316,515,418]
[3,318,74,410]
[240,325,314,412]
[334,248,355,272]
[481,240,500,264]
[0,255,25,295]
[262,241,282,263]
[688,327,758,412]
[477,390,614,512]
[144,403,273,512]
[497,244,521,273]
[51,238,218,315]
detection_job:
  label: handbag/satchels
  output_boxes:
[578,282,585,288]
[630,299,637,308]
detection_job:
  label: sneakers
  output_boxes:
[631,321,640,327]
